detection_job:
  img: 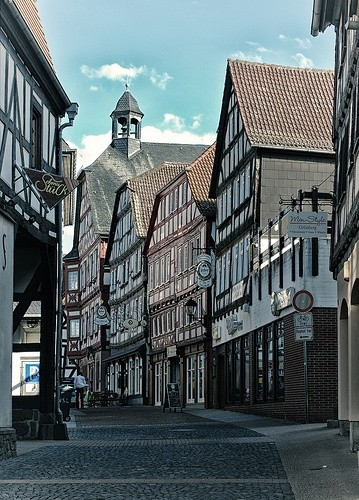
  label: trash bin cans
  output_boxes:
[60,386,74,422]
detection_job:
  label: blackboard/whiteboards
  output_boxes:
[164,383,182,409]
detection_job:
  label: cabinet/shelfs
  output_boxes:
[162,382,183,413]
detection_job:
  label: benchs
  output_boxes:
[88,392,119,408]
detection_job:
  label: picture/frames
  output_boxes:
[19,360,40,396]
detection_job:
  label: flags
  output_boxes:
[287,211,327,238]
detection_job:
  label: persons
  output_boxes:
[74,373,86,409]
